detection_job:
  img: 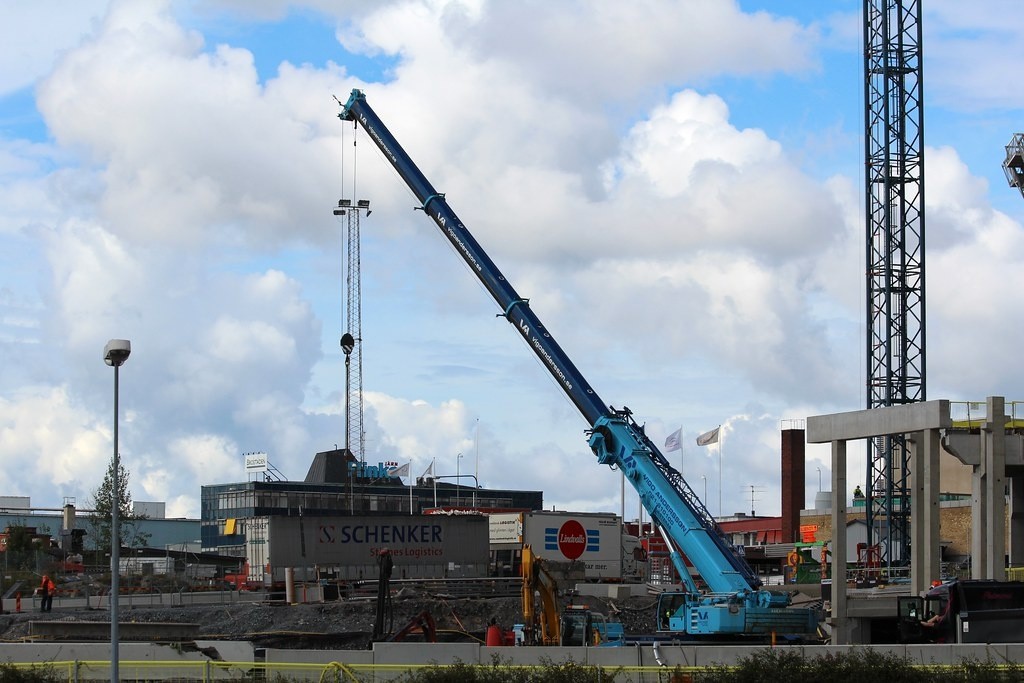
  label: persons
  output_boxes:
[377,548,394,578]
[40,576,54,612]
[854,486,863,498]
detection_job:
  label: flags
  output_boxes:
[390,463,409,476]
[426,462,432,476]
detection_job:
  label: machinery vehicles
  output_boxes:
[511,542,609,647]
[365,546,437,650]
[332,86,822,648]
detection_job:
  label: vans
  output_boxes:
[896,579,1024,644]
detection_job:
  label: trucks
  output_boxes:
[485,509,649,590]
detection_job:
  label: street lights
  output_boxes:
[102,337,131,683]
